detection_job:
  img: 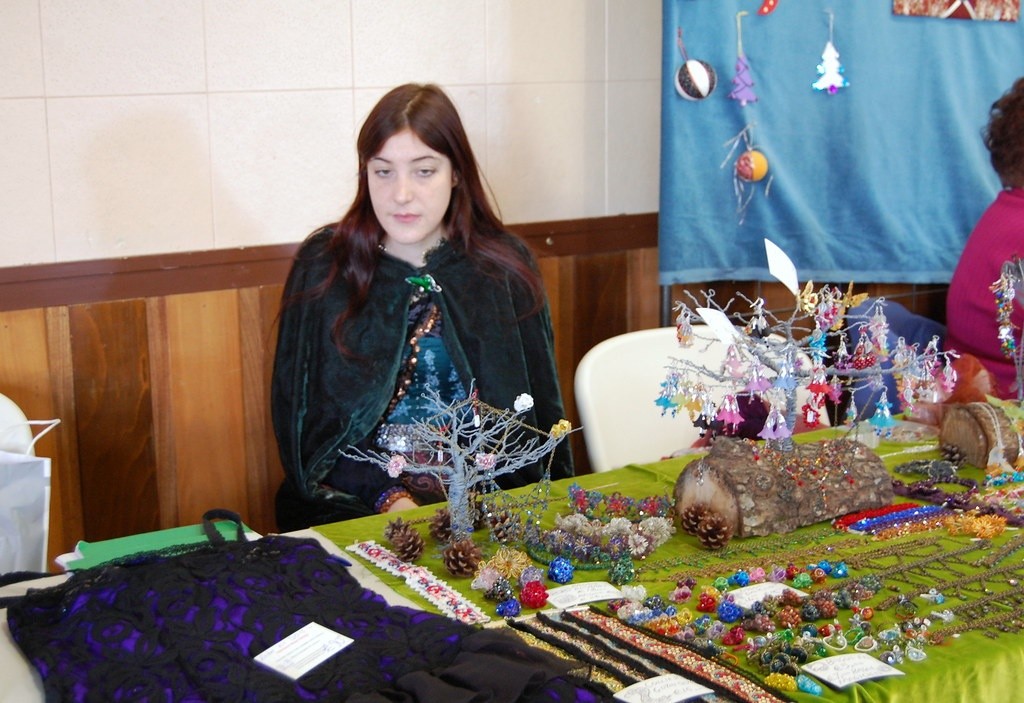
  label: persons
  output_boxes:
[946,76,1024,401]
[271,83,575,528]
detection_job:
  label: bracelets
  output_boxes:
[376,486,415,515]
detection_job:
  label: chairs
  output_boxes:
[843,294,948,420]
[571,323,840,475]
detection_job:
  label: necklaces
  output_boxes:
[426,398,558,543]
